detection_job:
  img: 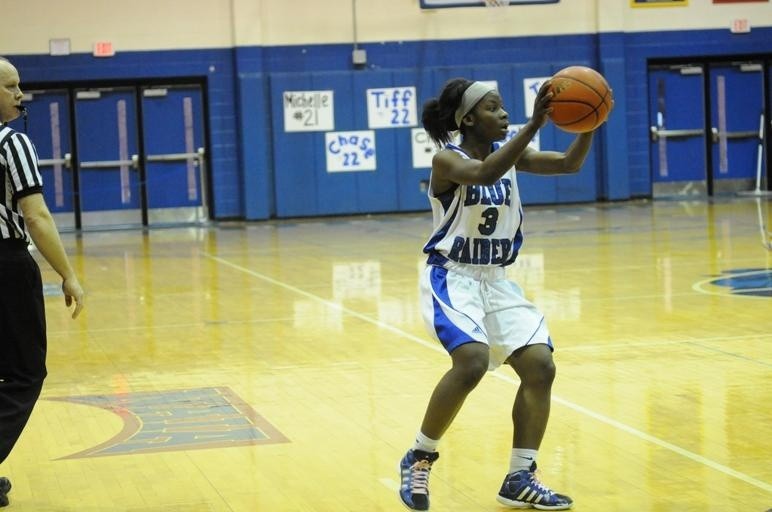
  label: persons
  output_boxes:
[398,77,615,511]
[0,56,85,508]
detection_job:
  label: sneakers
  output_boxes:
[399,448,439,512]
[497,461,573,510]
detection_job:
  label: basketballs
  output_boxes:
[548,65,611,133]
